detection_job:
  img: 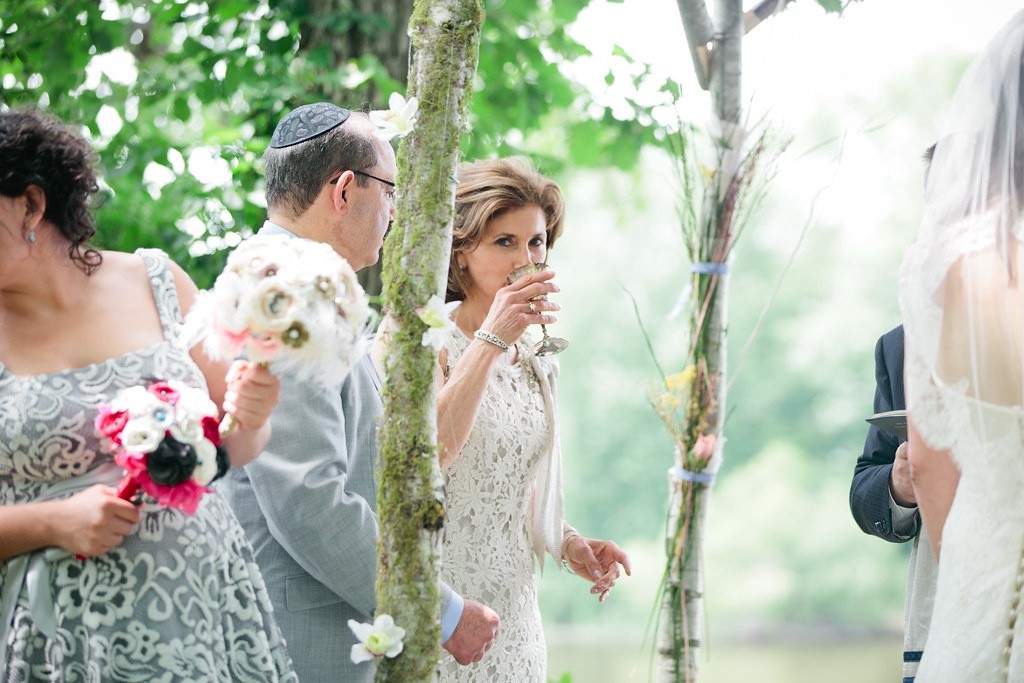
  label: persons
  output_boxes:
[372,152,632,682]
[210,102,499,680]
[902,8,1024,683]
[849,138,1000,681]
[0,111,302,683]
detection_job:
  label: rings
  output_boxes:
[528,301,537,314]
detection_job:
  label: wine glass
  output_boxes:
[506,264,569,356]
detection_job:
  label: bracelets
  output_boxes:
[561,528,580,575]
[473,329,511,352]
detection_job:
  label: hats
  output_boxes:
[269,102,349,148]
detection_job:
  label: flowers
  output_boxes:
[91,376,230,514]
[200,253,343,438]
